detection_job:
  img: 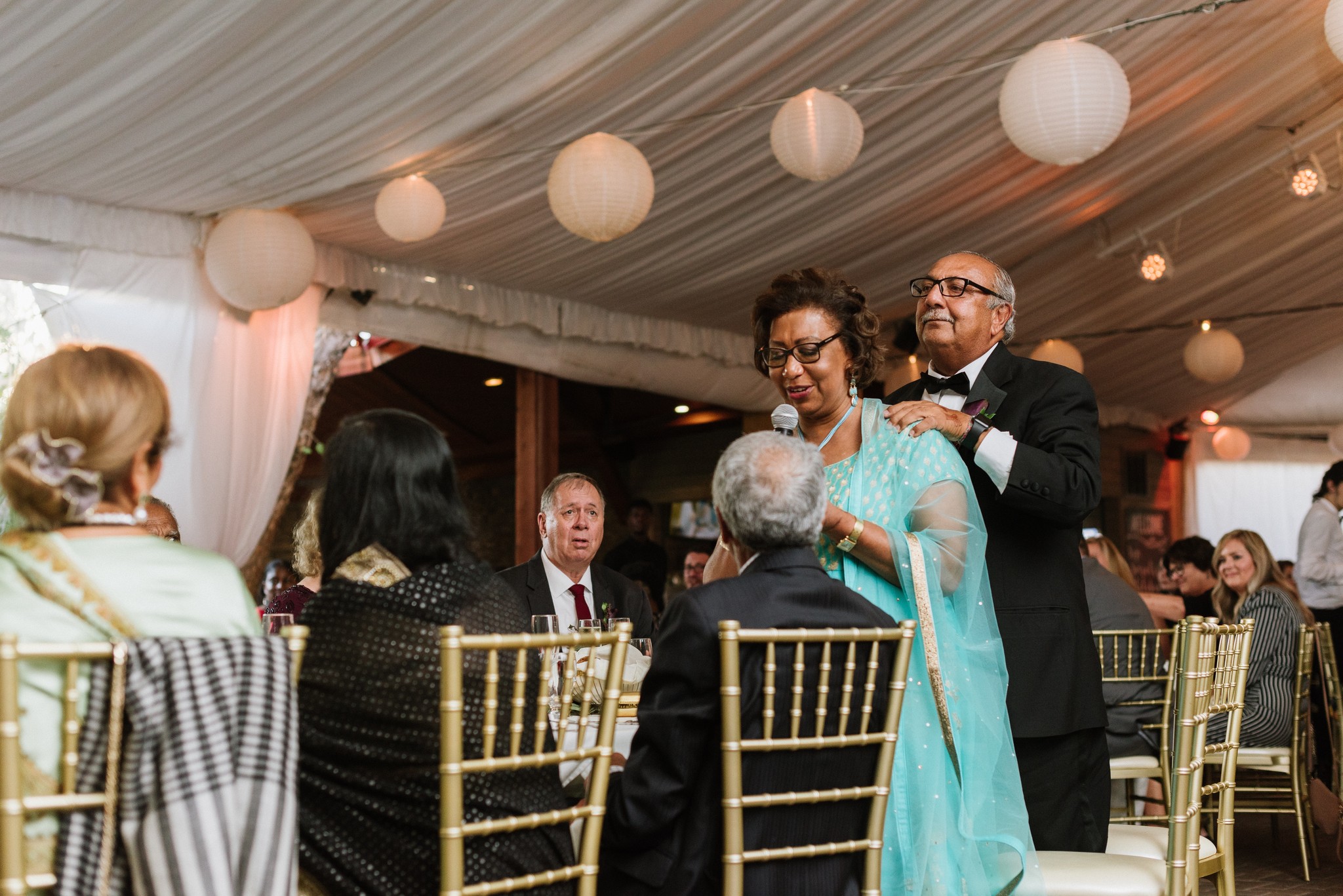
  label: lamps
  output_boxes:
[998,37,1133,166]
[1033,339,1086,372]
[204,206,314,310]
[1132,239,1174,285]
[544,130,655,242]
[1211,428,1251,460]
[1184,328,1244,383]
[374,178,447,243]
[770,87,863,185]
[1283,151,1330,201]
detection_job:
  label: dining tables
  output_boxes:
[546,701,641,796]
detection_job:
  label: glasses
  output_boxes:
[1165,564,1185,578]
[758,330,844,368]
[683,563,704,574]
[908,277,1006,300]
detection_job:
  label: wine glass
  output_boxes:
[630,638,654,658]
[261,613,295,640]
[609,618,630,635]
[579,618,603,651]
[532,614,561,704]
[545,649,577,723]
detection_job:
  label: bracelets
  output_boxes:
[719,533,731,553]
[958,413,979,445]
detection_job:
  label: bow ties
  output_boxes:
[919,370,970,397]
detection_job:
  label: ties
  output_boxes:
[569,584,593,628]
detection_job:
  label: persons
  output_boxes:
[1075,534,1169,761]
[493,470,658,658]
[275,407,584,896]
[581,433,901,896]
[884,249,1110,856]
[1290,457,1342,799]
[1144,529,1316,828]
[139,493,741,649]
[700,266,1039,895]
[0,341,266,896]
[1087,528,1301,694]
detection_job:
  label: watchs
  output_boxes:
[831,512,865,552]
[959,412,993,455]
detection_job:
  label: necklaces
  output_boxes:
[76,508,138,530]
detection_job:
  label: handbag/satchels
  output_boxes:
[1309,776,1343,835]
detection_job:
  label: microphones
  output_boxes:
[769,403,799,438]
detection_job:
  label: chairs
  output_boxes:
[1314,622,1343,863]
[1104,618,1255,896]
[438,620,633,896]
[718,620,917,896]
[1007,614,1220,896]
[1091,625,1171,828]
[0,624,309,896]
[1202,622,1318,881]
